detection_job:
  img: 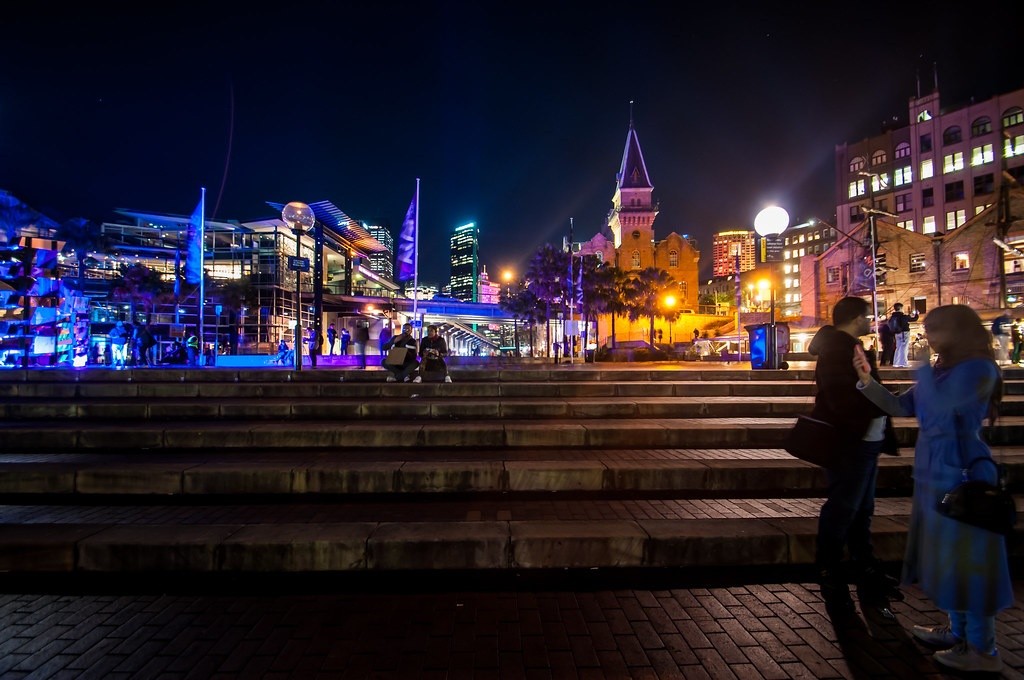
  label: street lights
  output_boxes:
[754,207,790,370]
[664,296,675,344]
[282,201,315,371]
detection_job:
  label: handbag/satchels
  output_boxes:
[935,457,1018,536]
[785,416,856,470]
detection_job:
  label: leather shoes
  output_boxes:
[827,597,855,616]
[862,602,896,624]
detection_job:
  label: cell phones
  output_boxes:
[855,344,869,372]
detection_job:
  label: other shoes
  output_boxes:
[413,376,422,383]
[404,376,410,382]
[911,625,959,648]
[386,375,397,383]
[444,375,453,383]
[934,642,1003,671]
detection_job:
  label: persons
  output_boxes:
[990,307,1024,366]
[808,297,902,601]
[852,304,1024,671]
[879,302,919,368]
[273,320,452,383]
[94,320,229,365]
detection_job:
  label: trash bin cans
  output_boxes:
[743,322,790,370]
